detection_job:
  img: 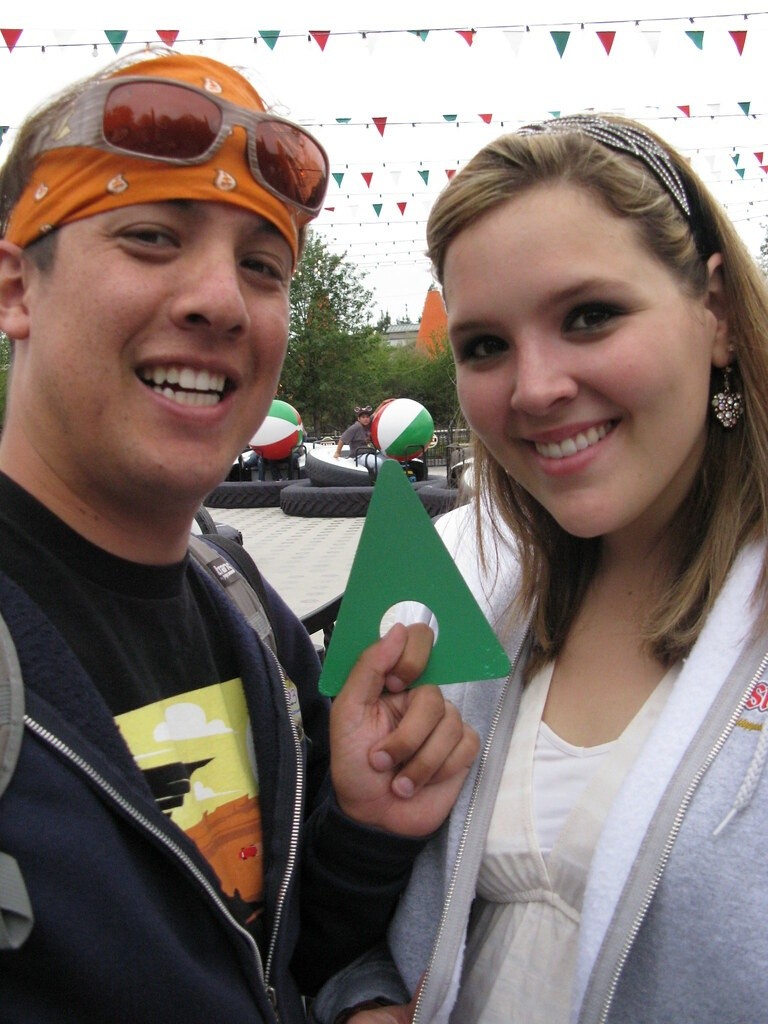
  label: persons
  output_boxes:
[0,53,482,1023]
[258,432,307,482]
[311,111,768,1023]
[333,405,384,475]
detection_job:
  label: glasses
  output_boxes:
[22,74,329,213]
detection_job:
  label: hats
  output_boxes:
[358,410,370,416]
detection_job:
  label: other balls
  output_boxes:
[247,399,305,460]
[370,398,435,462]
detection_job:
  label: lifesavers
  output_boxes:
[449,461,485,495]
[202,477,313,509]
[415,479,472,519]
[279,471,446,518]
[304,444,428,487]
